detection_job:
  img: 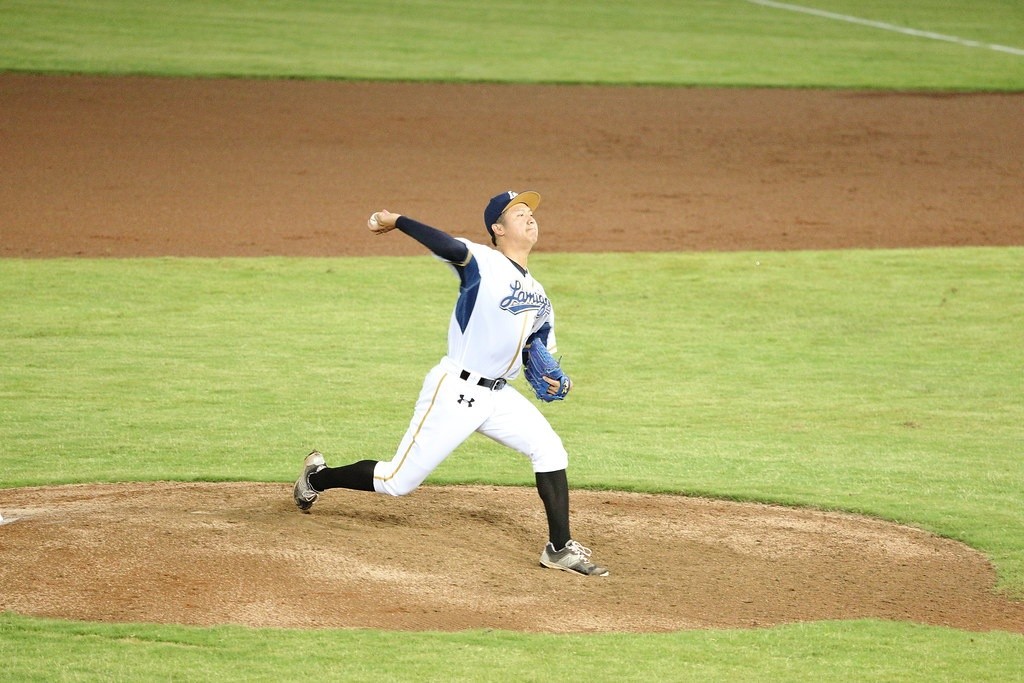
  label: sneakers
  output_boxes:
[293,451,326,510]
[539,540,610,577]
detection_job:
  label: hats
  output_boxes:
[484,190,541,235]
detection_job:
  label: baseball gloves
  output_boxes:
[524,337,571,404]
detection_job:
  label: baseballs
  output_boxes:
[370,212,385,226]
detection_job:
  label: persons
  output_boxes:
[293,191,609,577]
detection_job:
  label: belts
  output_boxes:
[460,370,507,392]
